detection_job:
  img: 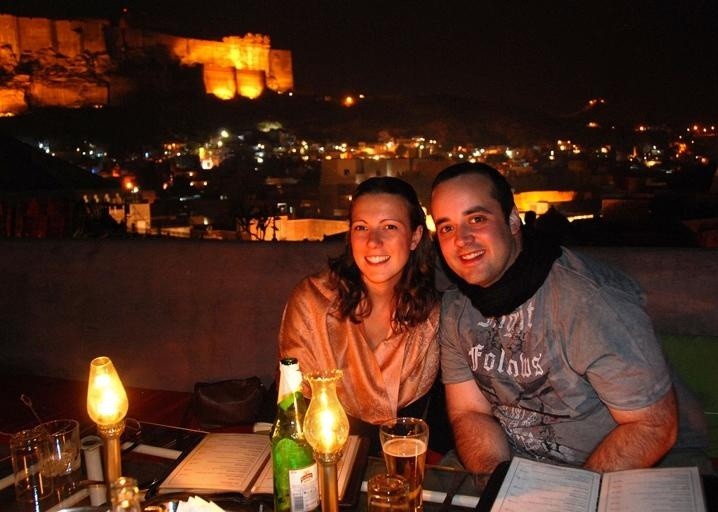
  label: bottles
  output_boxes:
[84,192,123,218]
[272,358,320,512]
[109,475,140,512]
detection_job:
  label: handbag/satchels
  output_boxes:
[182,375,266,438]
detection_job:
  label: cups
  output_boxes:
[8,420,79,501]
[366,417,429,512]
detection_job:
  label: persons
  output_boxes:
[428,161,679,495]
[252,176,447,478]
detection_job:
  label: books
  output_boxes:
[156,433,370,504]
[470,456,705,511]
